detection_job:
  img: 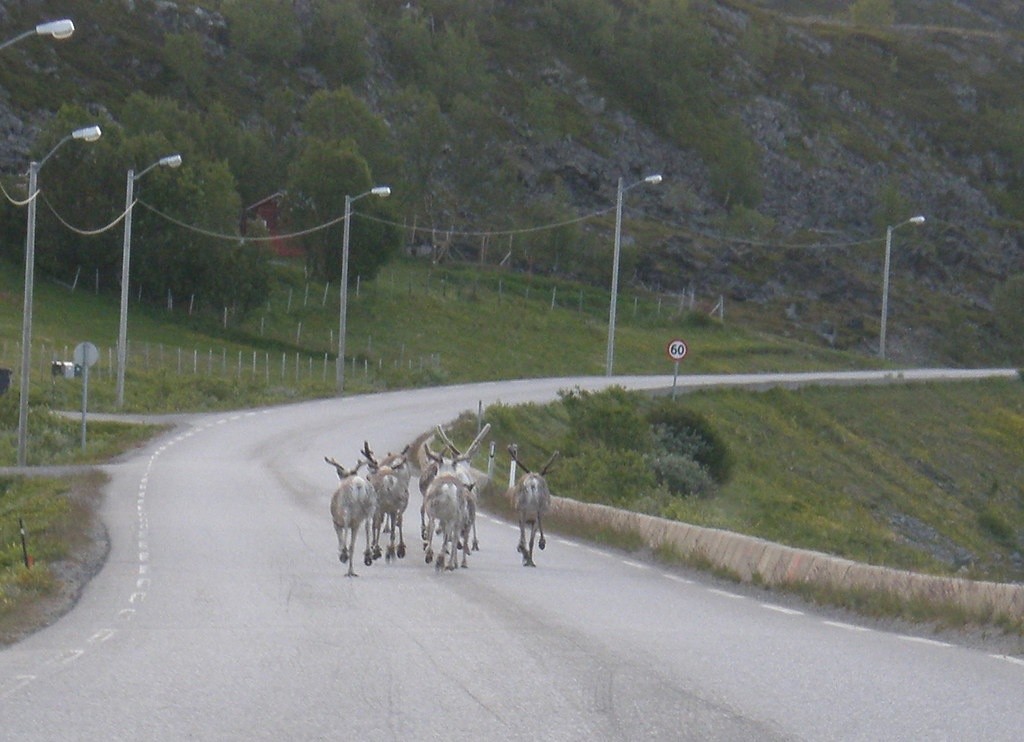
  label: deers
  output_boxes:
[324,441,411,580]
[505,445,559,567]
[419,421,491,572]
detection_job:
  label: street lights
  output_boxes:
[335,186,391,396]
[115,154,183,408]
[605,174,663,377]
[16,125,102,469]
[879,216,925,359]
